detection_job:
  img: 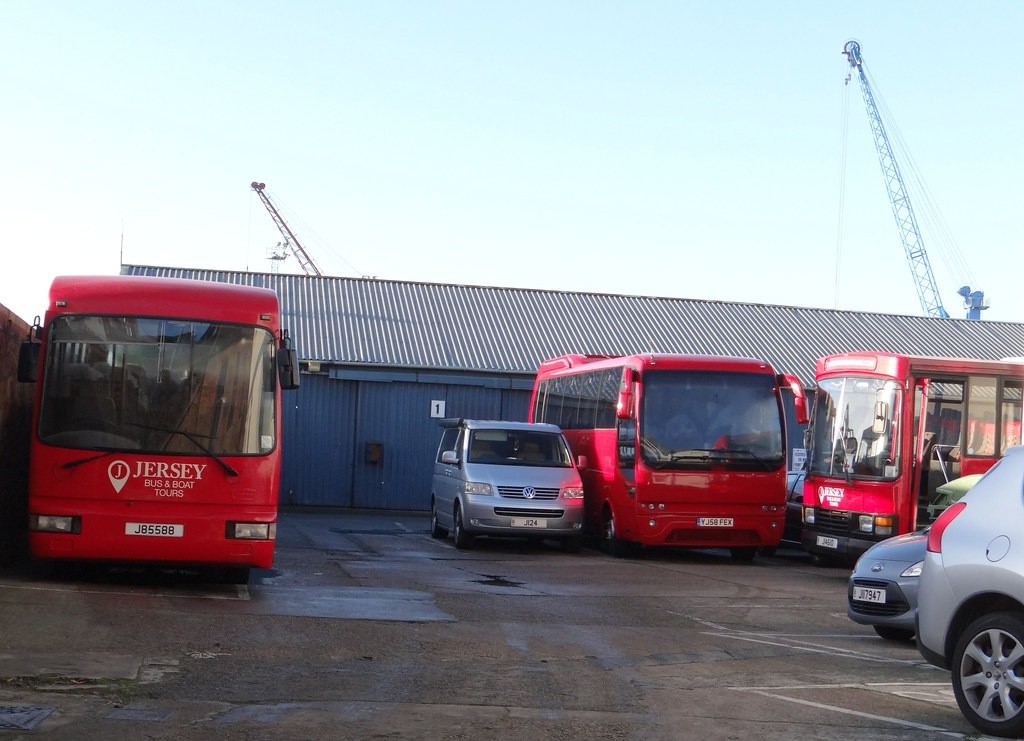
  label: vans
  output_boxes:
[426,412,588,556]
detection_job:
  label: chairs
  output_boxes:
[914,432,938,471]
[60,360,252,444]
[947,422,1022,462]
[518,441,546,460]
[471,441,497,458]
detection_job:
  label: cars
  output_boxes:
[847,469,983,641]
[912,440,1024,741]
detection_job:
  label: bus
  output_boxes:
[524,351,812,566]
[16,274,300,576]
[801,350,1024,564]
